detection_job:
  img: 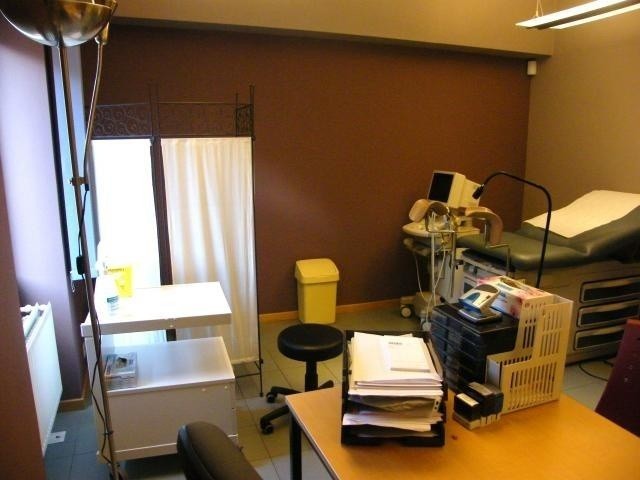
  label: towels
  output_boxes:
[522,187,640,239]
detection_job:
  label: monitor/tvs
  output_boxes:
[427,171,480,210]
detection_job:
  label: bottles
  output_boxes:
[107,281,119,316]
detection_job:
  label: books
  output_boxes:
[341,331,443,440]
[103,353,137,377]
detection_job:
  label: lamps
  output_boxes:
[513,0,640,38]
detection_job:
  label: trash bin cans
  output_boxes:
[295,258,340,324]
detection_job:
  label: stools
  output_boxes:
[257,323,346,436]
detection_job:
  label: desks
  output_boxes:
[282,382,640,478]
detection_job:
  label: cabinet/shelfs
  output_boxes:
[16,297,66,462]
[1,8,101,413]
[73,280,242,480]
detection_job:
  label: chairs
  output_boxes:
[591,316,640,440]
[175,419,265,480]
[454,187,640,371]
